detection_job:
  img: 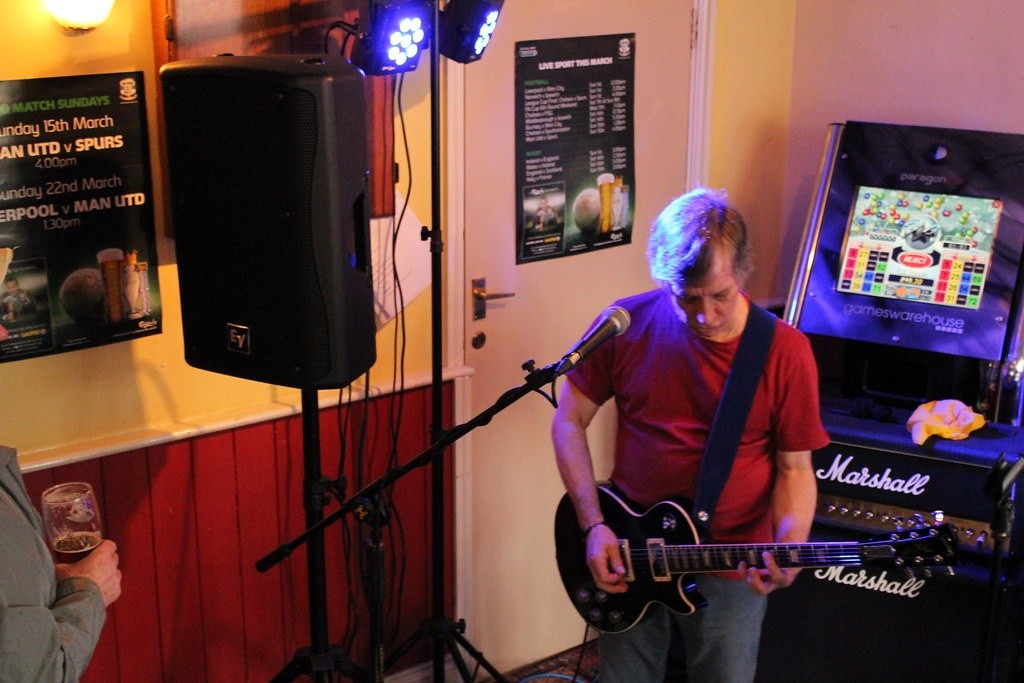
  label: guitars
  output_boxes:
[553,480,962,635]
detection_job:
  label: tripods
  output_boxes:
[269,389,371,683]
[374,0,509,683]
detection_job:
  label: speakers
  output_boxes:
[158,53,375,391]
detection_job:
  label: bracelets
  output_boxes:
[582,521,606,534]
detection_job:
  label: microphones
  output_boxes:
[556,306,630,377]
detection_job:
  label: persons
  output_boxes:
[0,277,36,321]
[548,188,830,682]
[0,445,123,683]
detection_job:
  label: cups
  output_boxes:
[41,482,102,564]
